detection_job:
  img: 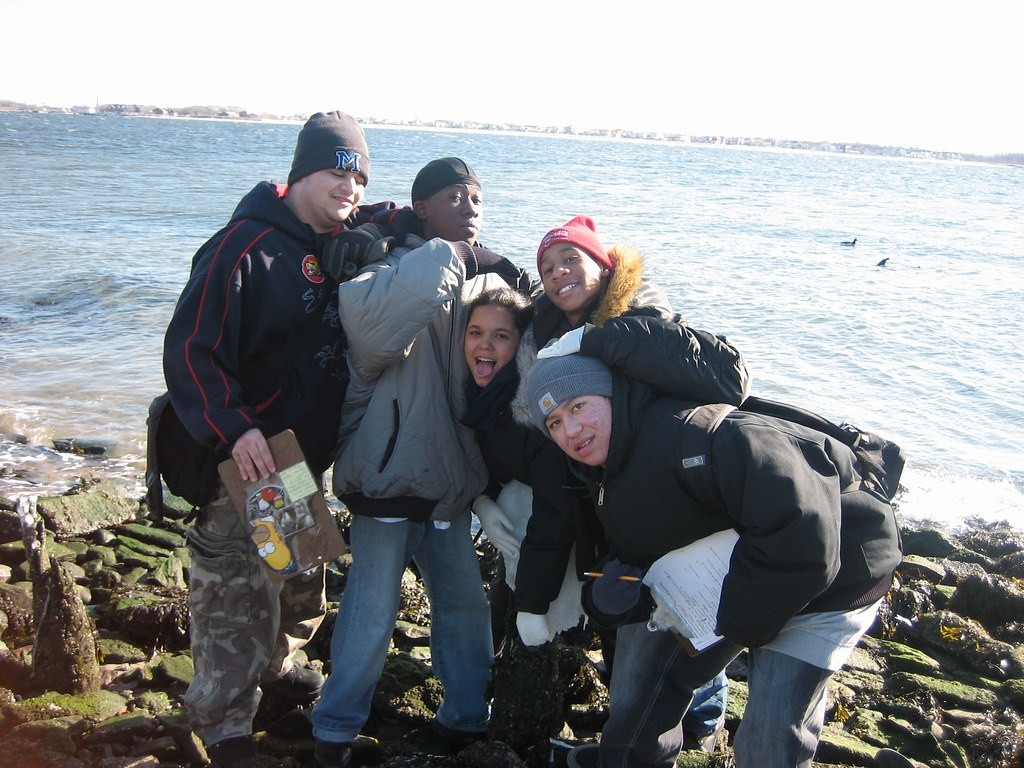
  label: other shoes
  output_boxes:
[208,734,256,768]
[313,738,355,768]
[257,666,327,698]
[682,728,721,754]
[565,742,601,768]
[431,717,488,748]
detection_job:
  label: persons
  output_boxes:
[161,109,413,767]
[310,154,521,768]
[463,215,751,768]
[521,351,903,768]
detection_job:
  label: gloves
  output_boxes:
[319,222,397,283]
[470,495,522,561]
[450,240,520,286]
[515,611,552,647]
[591,559,642,616]
[536,325,585,359]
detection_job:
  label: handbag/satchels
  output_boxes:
[144,393,223,524]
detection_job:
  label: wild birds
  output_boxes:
[840,238,858,246]
[876,258,889,267]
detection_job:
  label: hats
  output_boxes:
[523,337,612,443]
[536,215,613,281]
[286,110,371,186]
[411,157,481,206]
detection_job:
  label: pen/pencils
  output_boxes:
[583,571,641,582]
